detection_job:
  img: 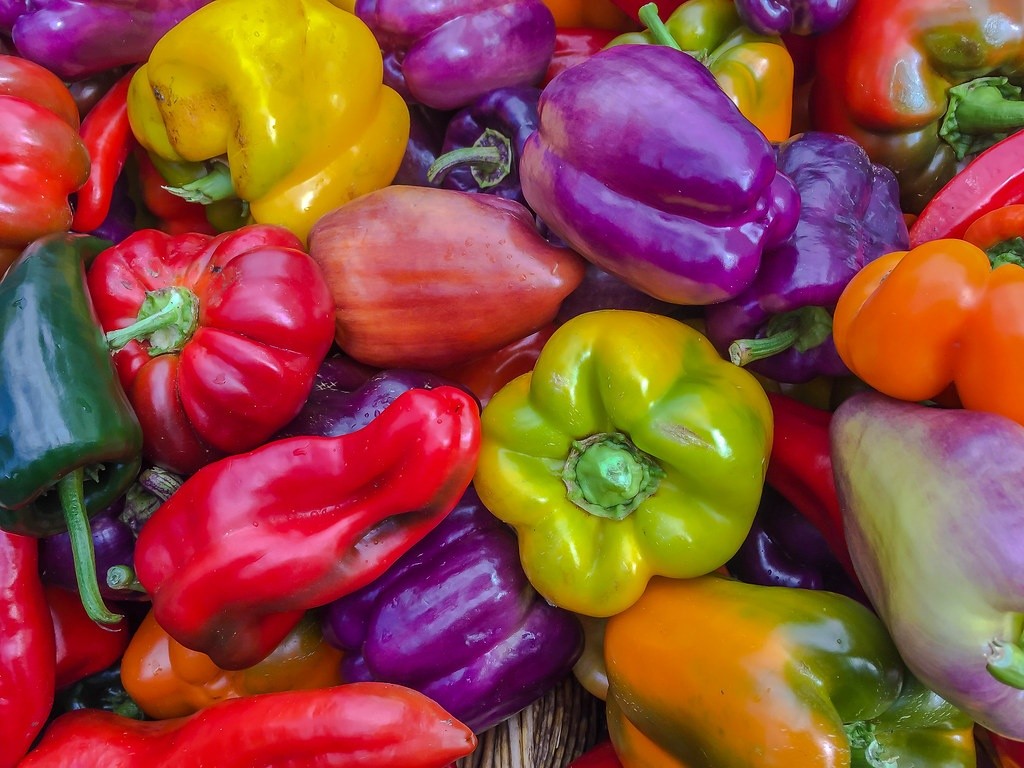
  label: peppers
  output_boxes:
[0,0,1024,768]
